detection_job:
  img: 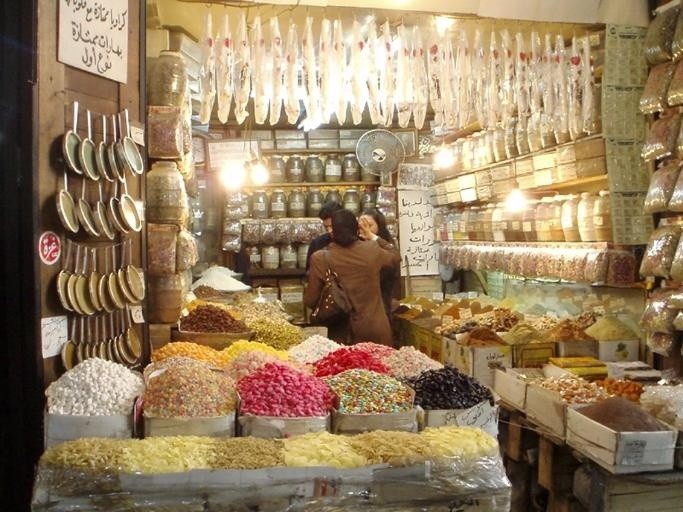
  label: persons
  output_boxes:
[357,208,400,321]
[305,202,344,275]
[302,210,401,349]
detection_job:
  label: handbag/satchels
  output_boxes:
[307,247,351,328]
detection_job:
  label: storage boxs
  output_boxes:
[333,408,418,436]
[431,333,443,360]
[417,327,431,355]
[566,404,679,474]
[557,342,597,359]
[539,437,573,493]
[495,367,552,409]
[141,408,236,440]
[526,383,606,437]
[507,415,538,461]
[416,384,500,440]
[443,338,510,386]
[513,345,555,367]
[237,413,332,441]
[42,406,133,447]
[597,340,640,363]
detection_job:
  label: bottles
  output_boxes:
[547,193,565,242]
[269,189,287,219]
[523,199,537,241]
[485,127,496,164]
[526,116,541,152]
[504,117,519,160]
[307,188,324,217]
[568,102,587,140]
[298,241,311,269]
[147,160,184,223]
[593,191,612,241]
[325,188,342,206]
[251,189,268,219]
[453,213,461,241]
[491,122,506,162]
[576,191,596,242]
[539,112,554,149]
[324,153,342,184]
[553,107,569,144]
[285,154,304,183]
[280,242,297,270]
[561,194,581,243]
[515,122,529,154]
[500,200,509,242]
[361,189,375,211]
[288,189,306,218]
[304,153,323,182]
[149,49,187,107]
[269,155,285,184]
[459,206,469,240]
[535,196,548,242]
[482,202,494,241]
[343,190,361,215]
[343,153,359,182]
[446,214,454,240]
[440,215,448,241]
[467,206,479,241]
[261,245,279,269]
[492,202,505,241]
[507,201,524,242]
[476,205,485,241]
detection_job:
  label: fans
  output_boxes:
[356,128,405,187]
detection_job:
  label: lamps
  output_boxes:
[506,183,525,212]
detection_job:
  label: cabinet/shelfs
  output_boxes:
[539,1,683,512]
[394,65,654,366]
[144,103,194,363]
[206,126,418,150]
[220,149,433,324]
[492,384,683,511]
